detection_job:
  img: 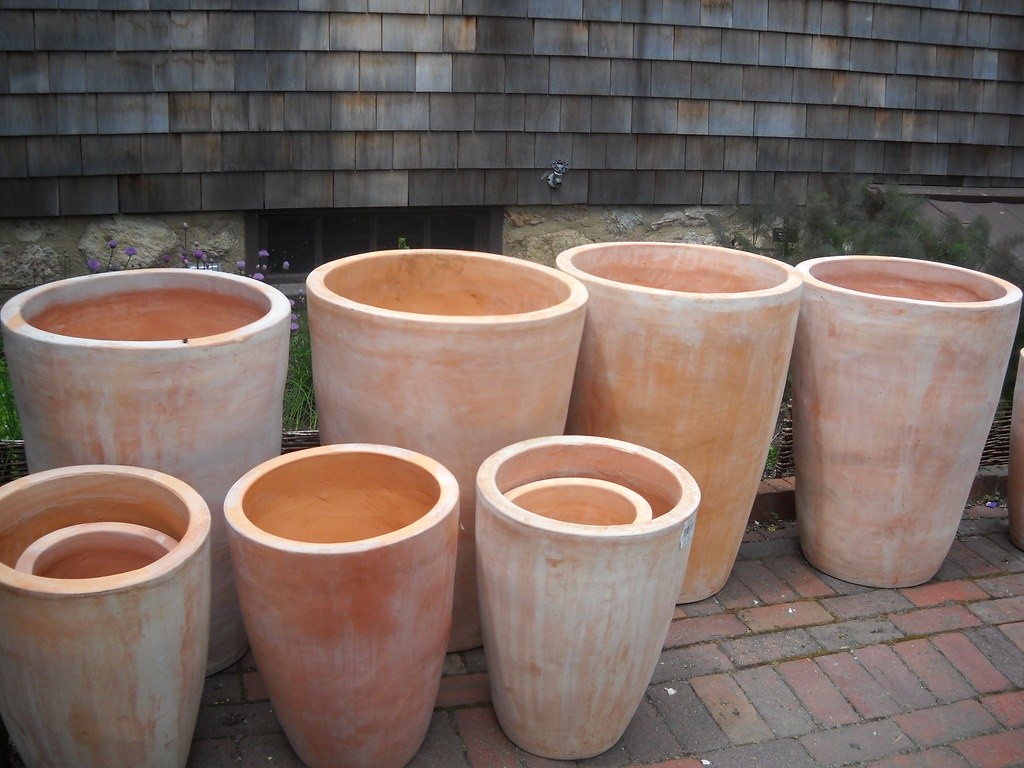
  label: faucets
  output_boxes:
[547,159,570,189]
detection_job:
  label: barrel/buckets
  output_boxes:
[502,477,653,524]
[307,248,588,652]
[556,242,799,604]
[476,435,701,760]
[1,267,292,677]
[1007,348,1024,550]
[224,443,461,768]
[14,522,179,579]
[0,464,212,768]
[793,254,1024,588]
